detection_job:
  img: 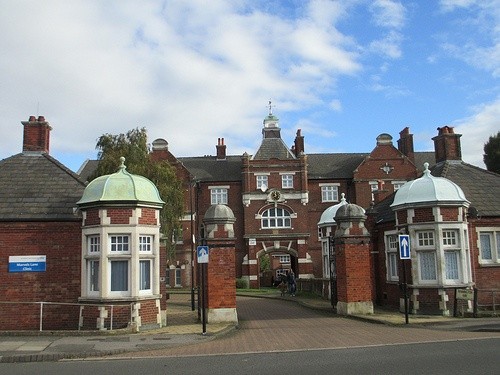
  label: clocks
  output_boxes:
[270,190,281,201]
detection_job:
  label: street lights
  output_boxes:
[188,175,198,310]
